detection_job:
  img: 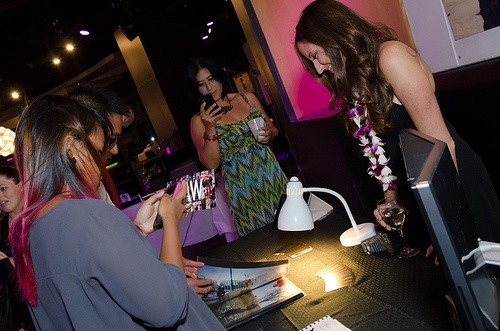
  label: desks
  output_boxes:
[189,201,459,331]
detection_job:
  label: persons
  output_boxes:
[0,84,229,331]
[295,0,500,264]
[188,58,290,237]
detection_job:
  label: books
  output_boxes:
[196,256,303,331]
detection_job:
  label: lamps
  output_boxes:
[80,21,92,36]
[276,176,378,248]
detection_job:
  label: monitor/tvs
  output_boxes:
[397,128,500,331]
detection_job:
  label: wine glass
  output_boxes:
[376,195,420,258]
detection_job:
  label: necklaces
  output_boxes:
[220,94,233,114]
[347,92,397,192]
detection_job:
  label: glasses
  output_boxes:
[107,135,120,149]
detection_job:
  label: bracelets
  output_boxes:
[203,133,219,141]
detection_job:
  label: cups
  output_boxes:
[246,117,266,141]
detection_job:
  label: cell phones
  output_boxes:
[153,181,178,229]
[199,94,221,116]
